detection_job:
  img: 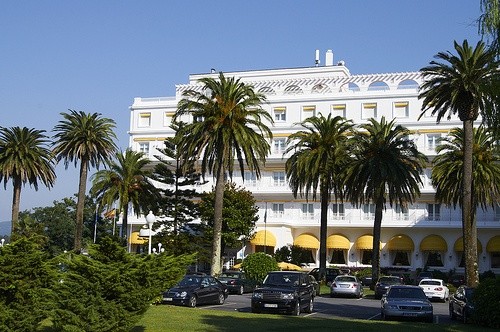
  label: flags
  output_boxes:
[104,208,115,219]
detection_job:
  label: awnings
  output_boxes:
[454,236,482,254]
[249,229,276,247]
[293,233,318,249]
[385,234,413,251]
[128,231,149,245]
[326,232,350,250]
[420,234,447,251]
[486,235,500,252]
[356,233,382,251]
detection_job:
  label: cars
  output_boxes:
[160,274,228,306]
[217,269,259,295]
[448,273,466,287]
[330,274,360,297]
[308,274,320,296]
[416,272,434,284]
[374,276,402,299]
[380,285,433,323]
[417,278,449,303]
[447,285,474,323]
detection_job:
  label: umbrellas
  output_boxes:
[277,261,301,271]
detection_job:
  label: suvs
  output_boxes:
[309,268,343,282]
[253,271,315,317]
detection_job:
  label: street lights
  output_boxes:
[145,210,155,253]
